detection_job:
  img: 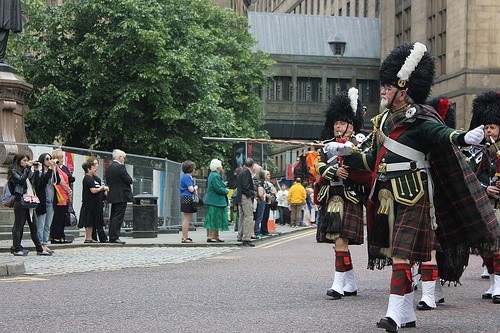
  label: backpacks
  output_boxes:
[1,179,16,207]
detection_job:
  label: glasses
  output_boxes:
[44,158,52,160]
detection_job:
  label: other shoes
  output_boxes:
[13,236,108,257]
[182,232,273,247]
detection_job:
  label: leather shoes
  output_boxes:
[327,289,342,299]
[380,317,399,333]
[400,321,416,328]
[342,290,357,296]
[482,275,490,279]
[493,295,500,303]
[416,301,431,310]
[482,293,492,299]
[109,239,126,244]
[438,298,444,303]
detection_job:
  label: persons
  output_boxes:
[236,158,258,246]
[77,156,109,243]
[323,48,485,333]
[315,96,373,298]
[287,178,306,228]
[48,150,72,243]
[49,163,75,240]
[231,163,266,238]
[416,91,500,310]
[179,161,199,243]
[35,153,58,253]
[6,154,52,256]
[260,170,278,236]
[276,183,289,226]
[105,149,135,245]
[203,159,230,243]
[301,181,318,228]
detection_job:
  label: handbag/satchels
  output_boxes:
[192,188,199,203]
[21,194,40,208]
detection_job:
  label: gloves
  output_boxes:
[324,141,354,156]
[464,124,484,145]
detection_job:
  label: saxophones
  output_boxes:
[338,114,383,182]
[490,136,500,185]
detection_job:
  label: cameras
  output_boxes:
[33,162,38,165]
[53,160,59,164]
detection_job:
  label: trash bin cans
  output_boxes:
[132,192,158,238]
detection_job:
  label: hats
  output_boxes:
[472,91,500,127]
[326,87,364,134]
[379,42,435,104]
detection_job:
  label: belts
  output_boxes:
[377,159,430,173]
